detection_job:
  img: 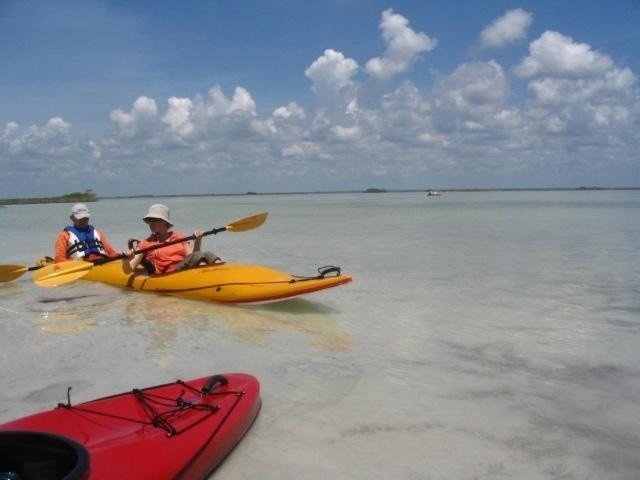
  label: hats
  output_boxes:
[143,204,174,228]
[72,204,90,220]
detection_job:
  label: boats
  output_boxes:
[61,280,352,357]
[0,372,262,480]
[37,252,352,305]
[427,191,441,196]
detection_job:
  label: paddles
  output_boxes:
[32,212,268,288]
[0,265,43,282]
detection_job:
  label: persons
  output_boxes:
[122,203,223,275]
[55,203,119,265]
